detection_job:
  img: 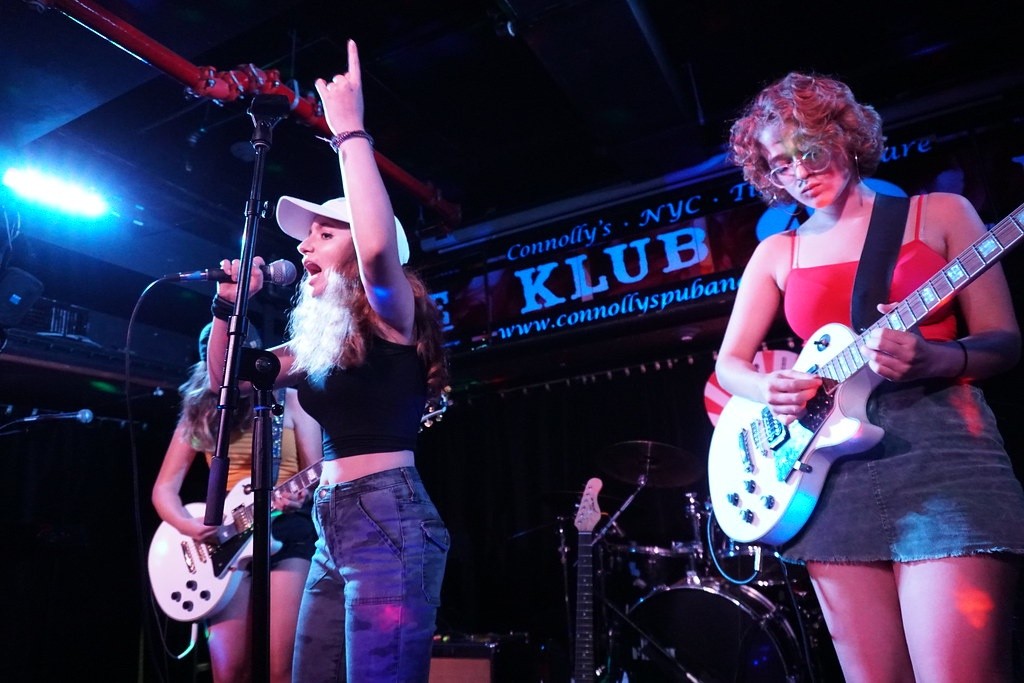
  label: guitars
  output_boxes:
[145,384,456,624]
[705,203,1024,549]
[569,476,604,683]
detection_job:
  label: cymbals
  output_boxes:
[597,439,705,488]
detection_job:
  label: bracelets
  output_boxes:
[327,130,374,152]
[208,291,236,323]
[953,337,968,374]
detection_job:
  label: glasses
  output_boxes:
[764,142,833,189]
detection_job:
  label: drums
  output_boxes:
[704,495,787,586]
[617,574,806,683]
[601,544,689,627]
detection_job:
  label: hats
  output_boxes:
[198,319,263,360]
[276,196,411,266]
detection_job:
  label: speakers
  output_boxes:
[429,632,532,683]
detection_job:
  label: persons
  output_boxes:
[207,38,452,683]
[152,321,322,683]
[714,73,1024,683]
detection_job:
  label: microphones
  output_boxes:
[161,259,297,286]
[16,408,93,425]
